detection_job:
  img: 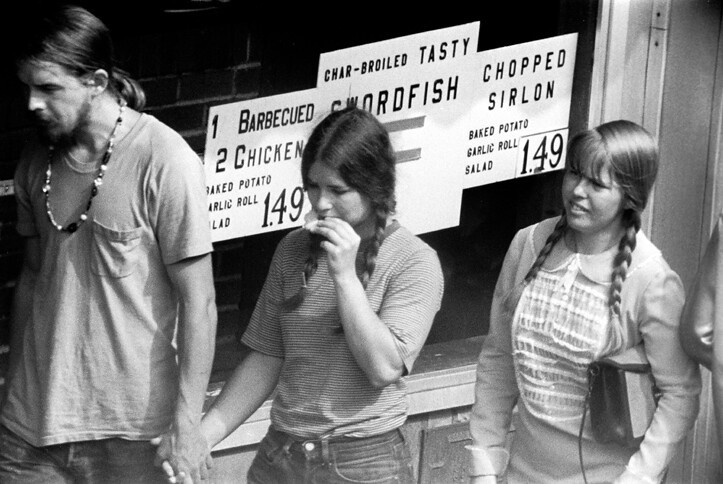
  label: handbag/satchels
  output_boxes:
[577,341,662,484]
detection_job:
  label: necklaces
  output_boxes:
[42,96,128,233]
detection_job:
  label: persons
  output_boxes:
[150,108,445,484]
[678,206,723,455]
[464,120,703,484]
[0,5,212,484]
